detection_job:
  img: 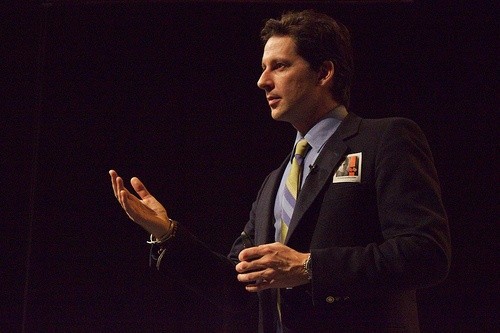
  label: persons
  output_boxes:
[108,10,449,333]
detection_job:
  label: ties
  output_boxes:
[277,138,311,333]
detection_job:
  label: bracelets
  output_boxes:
[146,219,177,242]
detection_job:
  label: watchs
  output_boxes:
[303,254,311,285]
